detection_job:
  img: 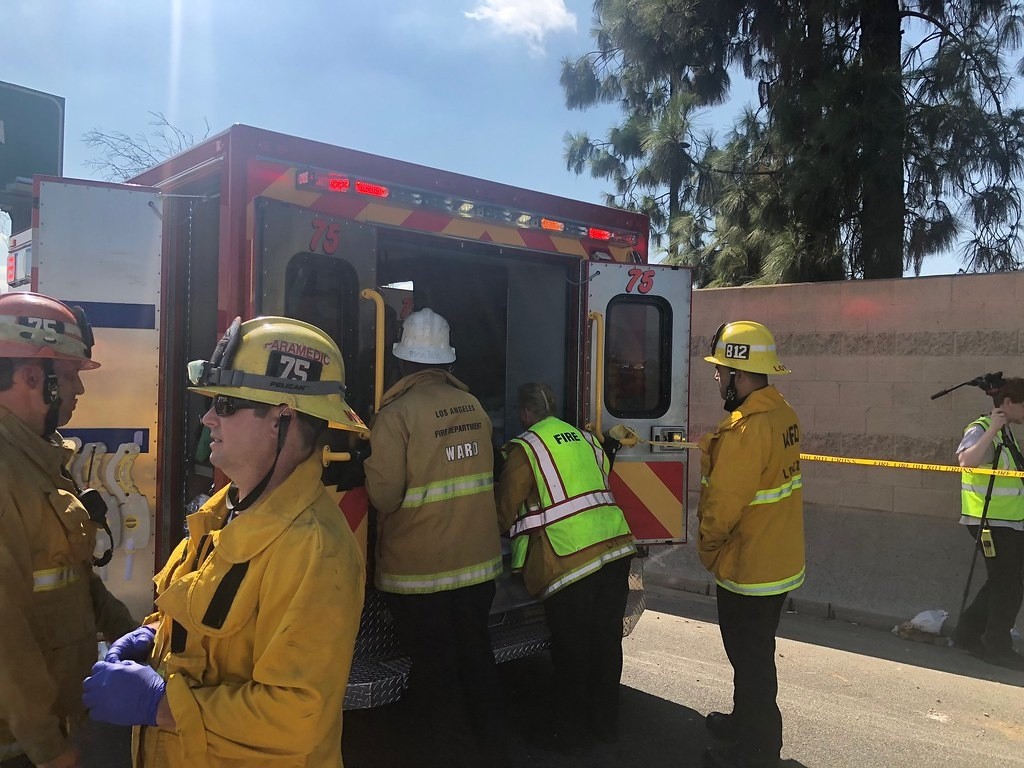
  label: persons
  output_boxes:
[0,293,145,768]
[83,314,366,768]
[951,377,1024,672]
[495,382,638,757]
[363,307,502,768]
[696,320,805,768]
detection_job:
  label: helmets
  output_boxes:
[0,290,100,369]
[392,307,456,364]
[187,317,370,433]
[704,320,791,375]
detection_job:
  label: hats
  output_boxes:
[512,382,556,411]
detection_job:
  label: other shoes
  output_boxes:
[707,744,778,768]
[706,711,738,740]
[595,730,616,741]
[528,723,592,755]
[985,650,1023,670]
[956,630,985,655]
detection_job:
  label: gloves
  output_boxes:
[81,659,168,727]
[97,627,157,662]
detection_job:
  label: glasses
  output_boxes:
[211,394,267,416]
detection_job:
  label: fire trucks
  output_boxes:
[8,124,695,733]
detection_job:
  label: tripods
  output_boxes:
[930,372,1024,640]
